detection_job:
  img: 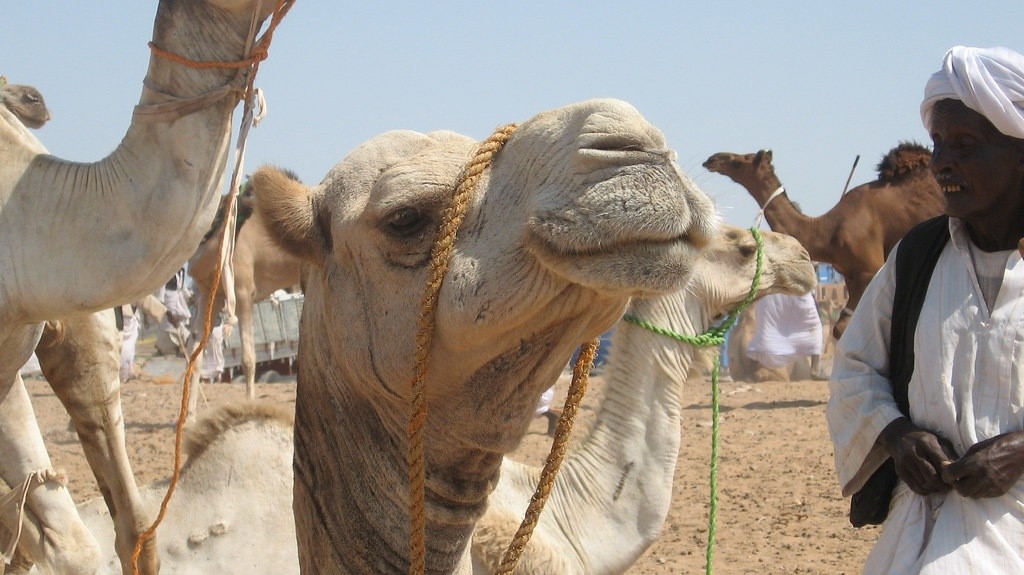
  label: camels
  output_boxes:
[22,98,821,575]
[703,140,949,333]
[0,1,299,575]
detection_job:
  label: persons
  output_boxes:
[112,266,197,385]
[826,44,1024,575]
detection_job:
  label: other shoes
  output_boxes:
[811,372,831,381]
[739,376,754,387]
[175,345,180,358]
[547,409,562,436]
[151,349,163,357]
